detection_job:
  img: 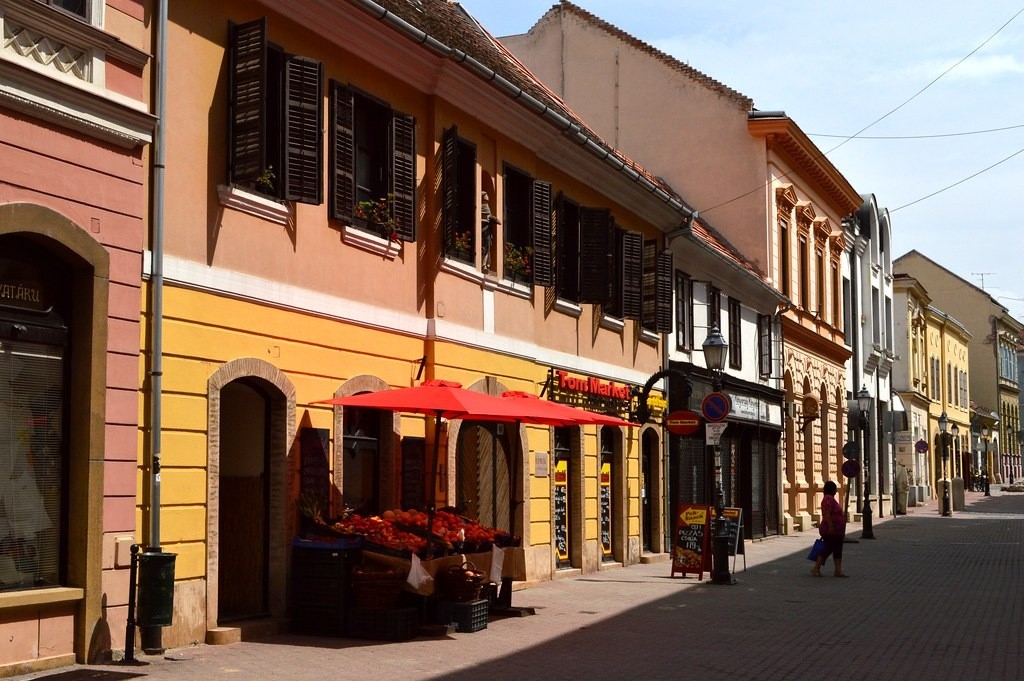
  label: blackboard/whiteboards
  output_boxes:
[710,506,742,557]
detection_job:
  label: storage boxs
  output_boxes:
[352,607,419,642]
[291,605,350,638]
[438,599,489,633]
[293,576,353,606]
[480,586,498,608]
[294,548,357,578]
[331,506,521,560]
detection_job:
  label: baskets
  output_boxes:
[441,561,486,602]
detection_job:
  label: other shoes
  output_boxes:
[811,570,823,577]
[834,573,847,577]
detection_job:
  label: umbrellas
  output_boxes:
[307,380,642,558]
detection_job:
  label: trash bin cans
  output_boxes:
[136,552,177,627]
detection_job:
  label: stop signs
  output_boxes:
[666,411,702,435]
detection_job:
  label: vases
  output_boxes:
[515,273,529,286]
[448,250,458,257]
[458,252,472,262]
[504,269,514,281]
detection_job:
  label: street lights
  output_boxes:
[937,411,953,517]
[701,321,739,586]
[1006,423,1014,484]
[981,423,992,496]
[857,383,877,540]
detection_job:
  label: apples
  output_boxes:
[330,514,435,553]
[382,508,520,546]
[464,570,480,577]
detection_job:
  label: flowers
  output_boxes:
[504,242,534,276]
[450,230,473,252]
[352,192,401,241]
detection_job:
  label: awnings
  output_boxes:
[848,389,909,431]
[974,442,997,452]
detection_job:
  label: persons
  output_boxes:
[972,466,989,492]
[810,481,849,578]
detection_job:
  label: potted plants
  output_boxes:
[240,161,276,194]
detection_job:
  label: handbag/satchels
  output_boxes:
[807,537,827,566]
[405,553,434,590]
